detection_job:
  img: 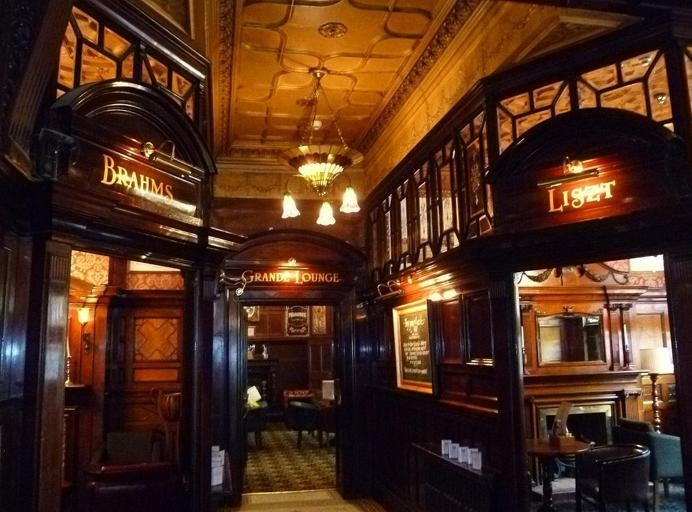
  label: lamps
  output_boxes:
[567,160,583,175]
[280,63,365,227]
[636,342,676,435]
[77,307,92,354]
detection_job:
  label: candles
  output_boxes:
[67,336,73,357]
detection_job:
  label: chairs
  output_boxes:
[89,427,163,468]
[80,463,172,512]
[289,402,321,447]
[574,445,653,512]
[613,418,685,496]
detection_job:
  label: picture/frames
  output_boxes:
[392,299,440,400]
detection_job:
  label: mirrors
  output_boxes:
[534,312,609,369]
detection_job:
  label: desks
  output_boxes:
[525,439,590,512]
[242,401,269,447]
